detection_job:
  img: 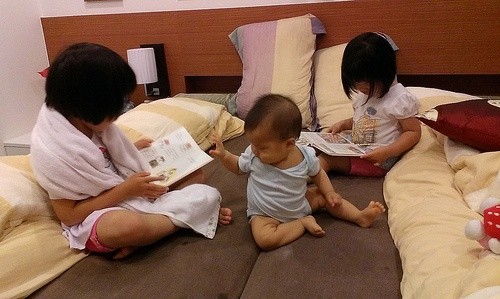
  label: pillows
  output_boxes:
[314,41,356,128]
[414,99,500,153]
[227,13,327,131]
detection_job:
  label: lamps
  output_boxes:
[127,47,158,97]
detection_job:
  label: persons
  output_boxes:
[208,93,386,252]
[28,40,232,260]
[304,30,422,186]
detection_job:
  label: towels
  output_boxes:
[30,101,222,250]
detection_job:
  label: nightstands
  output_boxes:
[2,130,32,156]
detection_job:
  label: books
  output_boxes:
[298,131,366,157]
[135,126,214,194]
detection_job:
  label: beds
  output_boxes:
[1,0,500,299]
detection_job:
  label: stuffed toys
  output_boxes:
[464,195,499,255]
